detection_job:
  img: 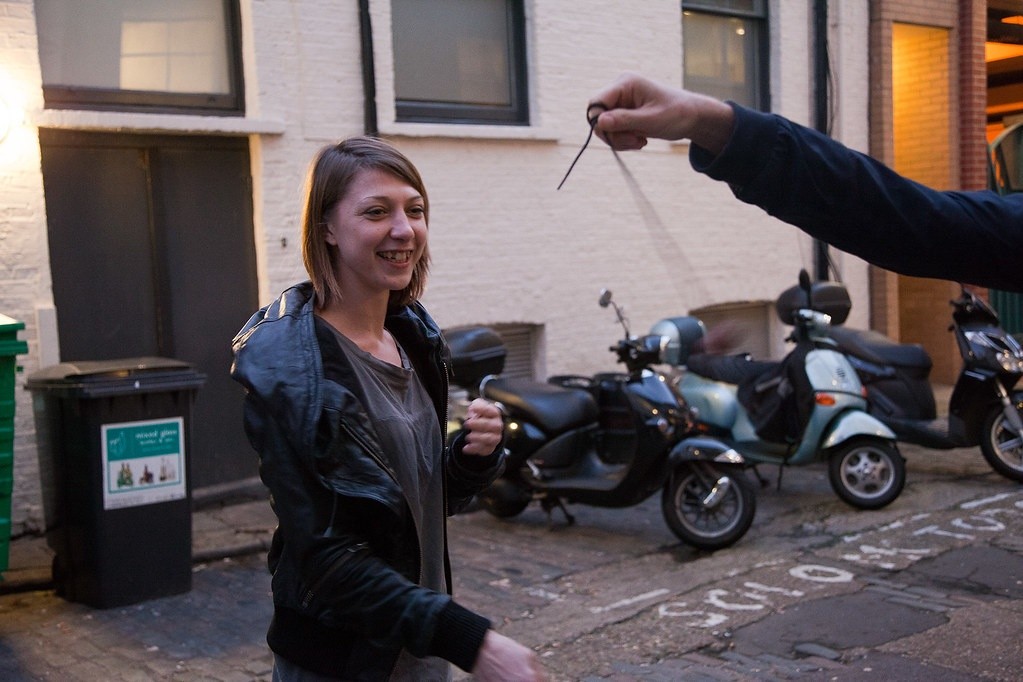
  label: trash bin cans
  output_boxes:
[0,312,28,583]
[22,354,211,610]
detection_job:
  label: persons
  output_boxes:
[230,137,553,682]
[581,77,1023,296]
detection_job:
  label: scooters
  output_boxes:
[647,265,907,511]
[441,288,759,553]
[803,265,1023,491]
